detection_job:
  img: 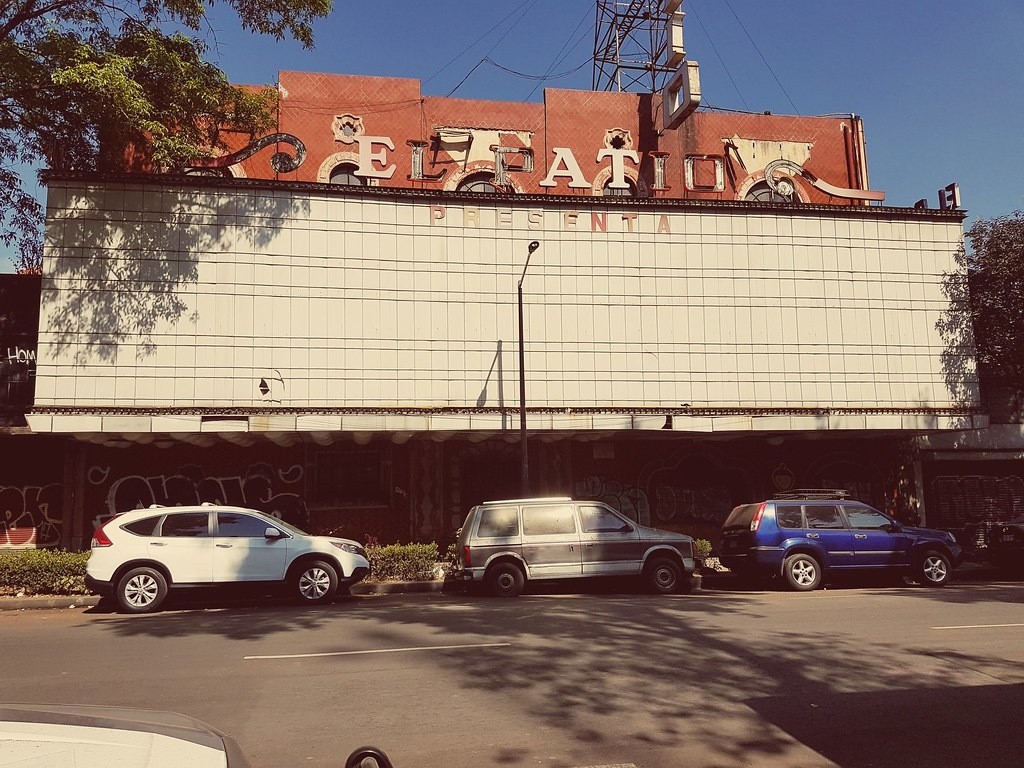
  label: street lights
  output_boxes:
[517,241,541,498]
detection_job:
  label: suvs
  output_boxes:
[718,488,961,591]
[84,502,374,614]
[454,497,698,596]
